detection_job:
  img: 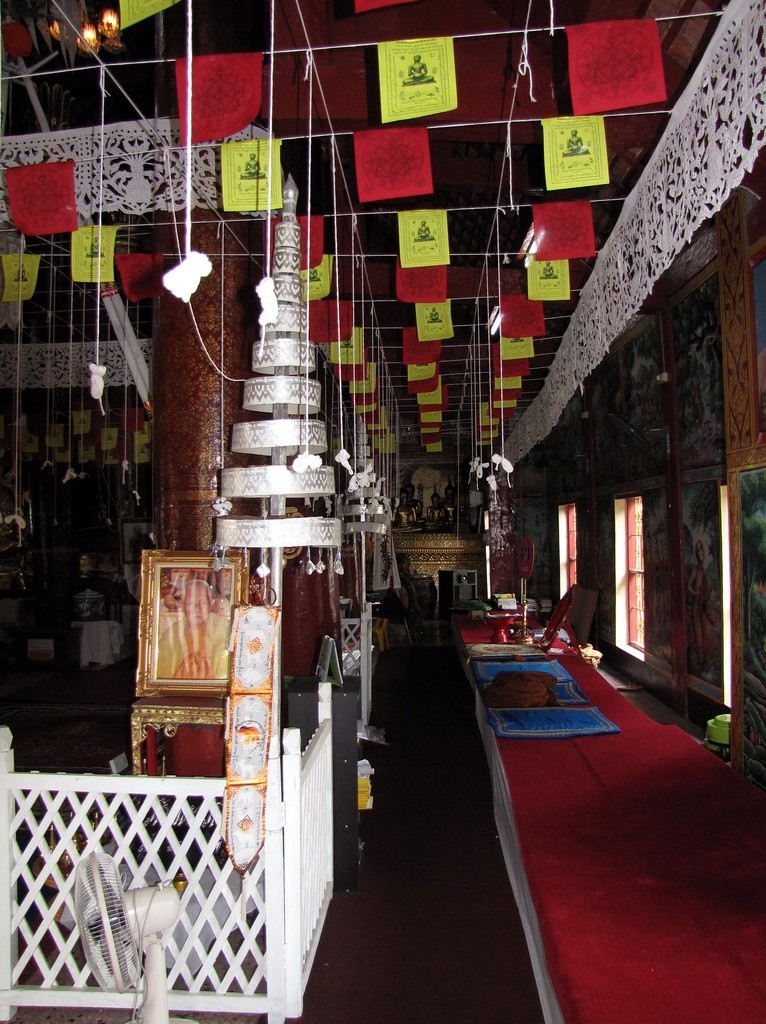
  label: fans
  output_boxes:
[607,412,654,452]
[74,851,198,1024]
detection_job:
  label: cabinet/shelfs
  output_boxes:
[438,568,477,619]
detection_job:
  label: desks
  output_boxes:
[129,704,224,775]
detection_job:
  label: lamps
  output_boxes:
[516,218,546,270]
[50,9,123,56]
[488,305,503,335]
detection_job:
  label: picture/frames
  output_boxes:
[136,549,248,701]
